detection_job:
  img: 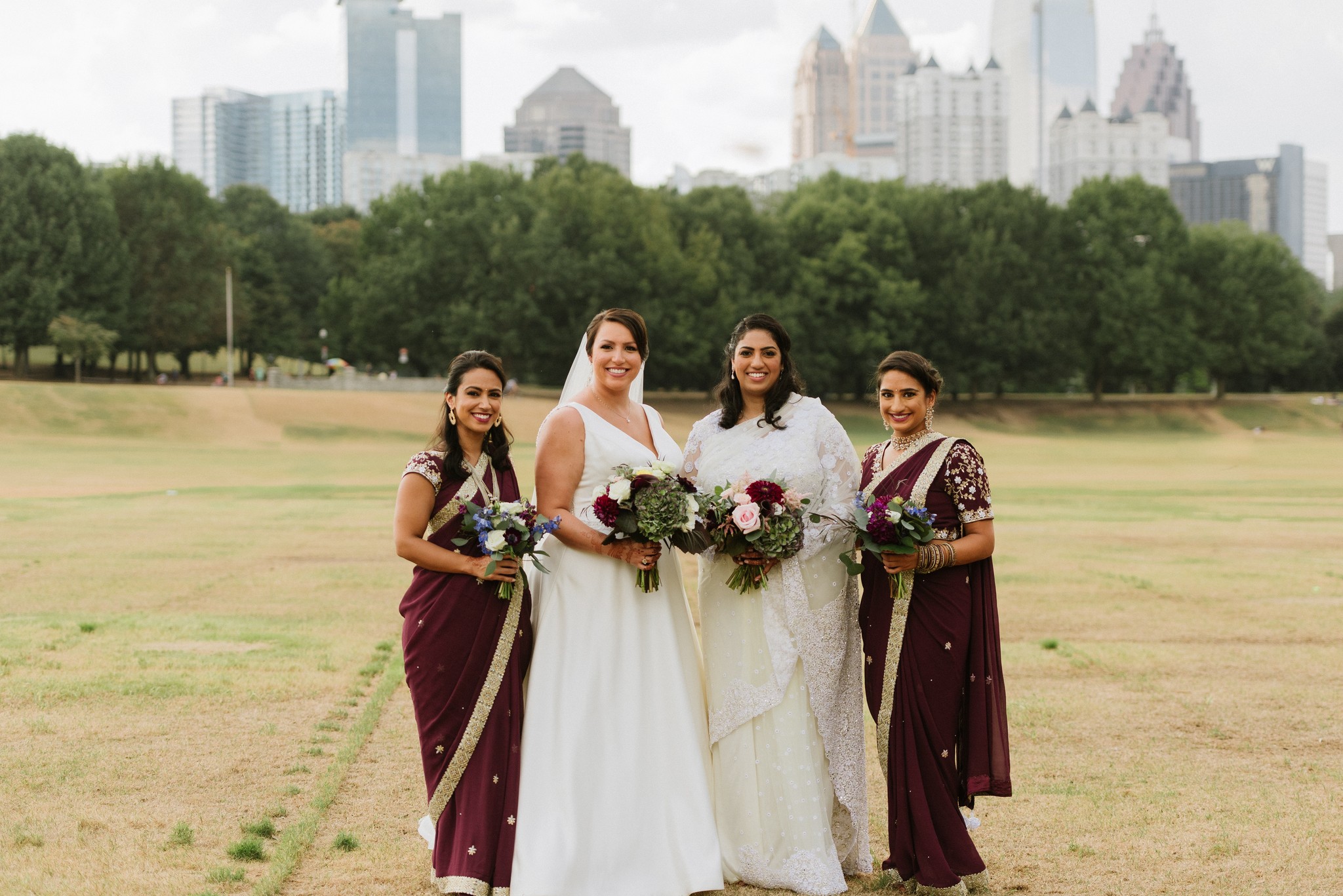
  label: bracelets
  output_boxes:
[915,542,957,574]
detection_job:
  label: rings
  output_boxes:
[642,556,648,565]
[766,559,770,564]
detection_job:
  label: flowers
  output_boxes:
[696,477,810,593]
[456,493,559,599]
[589,463,702,593]
[841,487,939,601]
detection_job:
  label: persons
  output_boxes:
[857,350,994,896]
[679,312,870,889]
[520,307,702,896]
[396,350,536,896]
[146,359,521,397]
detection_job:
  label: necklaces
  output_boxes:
[460,454,500,509]
[889,429,928,449]
[591,383,631,424]
[741,410,751,422]
